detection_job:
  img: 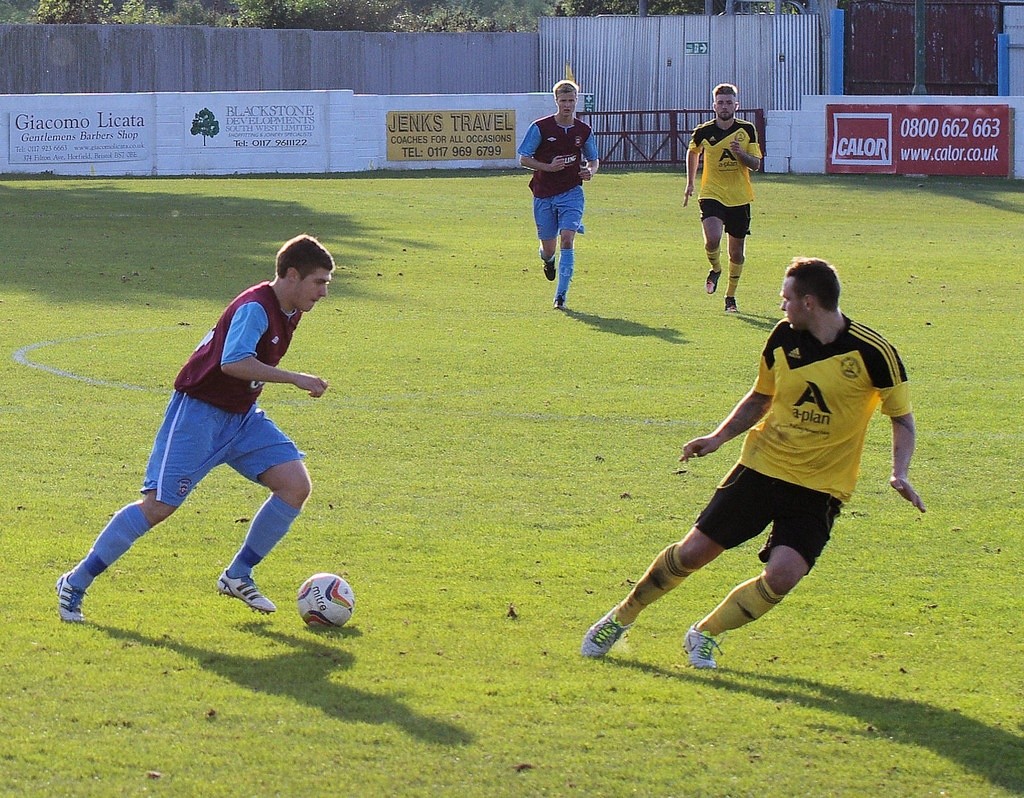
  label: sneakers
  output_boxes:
[544,258,556,282]
[552,294,566,310]
[705,268,722,294]
[215,566,277,615]
[683,621,724,670]
[725,294,738,314]
[53,572,87,623]
[582,608,636,659]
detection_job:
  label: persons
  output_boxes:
[682,84,764,313]
[581,259,926,668]
[518,80,599,308]
[55,236,336,622]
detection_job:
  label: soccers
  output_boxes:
[296,572,355,629]
